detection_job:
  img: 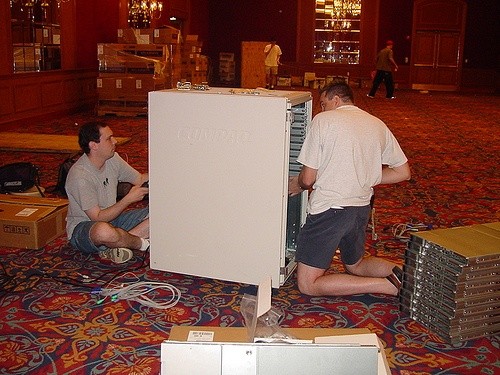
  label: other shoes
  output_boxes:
[98,248,133,263]
[386,265,403,288]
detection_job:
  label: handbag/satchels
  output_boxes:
[0,161,39,195]
[54,159,79,195]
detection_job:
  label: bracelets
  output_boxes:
[300,186,305,189]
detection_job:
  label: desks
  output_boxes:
[0,133,132,161]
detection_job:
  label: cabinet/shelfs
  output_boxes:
[314,0,360,65]
[0,72,96,125]
[410,0,467,90]
[8,0,63,73]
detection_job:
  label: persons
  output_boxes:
[65,121,151,263]
[288,80,412,296]
[367,40,398,99]
[264,38,282,89]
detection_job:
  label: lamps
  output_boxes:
[127,0,163,29]
[324,0,362,31]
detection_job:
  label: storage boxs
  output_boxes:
[0,193,69,251]
[161,323,390,375]
[12,47,41,61]
[13,60,40,71]
[36,28,61,45]
[96,29,349,101]
